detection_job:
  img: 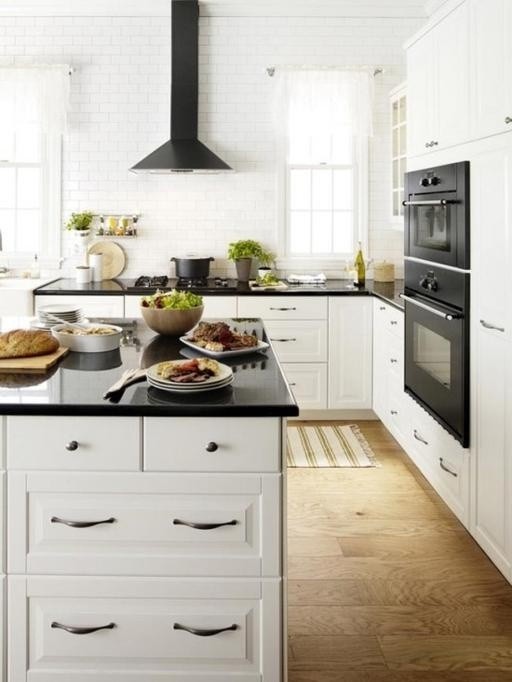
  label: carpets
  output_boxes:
[284,423,381,469]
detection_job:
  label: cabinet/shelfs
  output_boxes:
[388,84,404,230]
[473,0,512,140]
[0,405,298,682]
[401,396,467,519]
[370,292,402,446]
[34,296,126,319]
[236,296,325,411]
[402,0,472,157]
[470,142,512,587]
[126,296,234,319]
[327,295,373,411]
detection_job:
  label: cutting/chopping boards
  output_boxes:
[0,346,72,369]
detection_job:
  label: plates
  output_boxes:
[178,348,268,368]
[180,335,270,359]
[147,386,234,406]
[146,359,235,393]
[30,318,90,330]
[38,304,82,326]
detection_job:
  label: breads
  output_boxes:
[0,330,58,358]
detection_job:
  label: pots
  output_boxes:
[170,256,215,278]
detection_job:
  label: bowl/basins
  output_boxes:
[59,348,123,372]
[141,306,204,338]
[51,322,123,353]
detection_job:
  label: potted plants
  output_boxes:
[64,212,94,245]
[229,239,276,282]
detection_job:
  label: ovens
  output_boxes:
[403,160,470,449]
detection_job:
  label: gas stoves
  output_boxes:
[125,275,237,319]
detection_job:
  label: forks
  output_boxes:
[108,368,139,394]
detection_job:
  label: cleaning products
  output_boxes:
[30,254,40,278]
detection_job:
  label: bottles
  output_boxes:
[354,241,366,287]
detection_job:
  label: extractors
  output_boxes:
[129,0,234,179]
